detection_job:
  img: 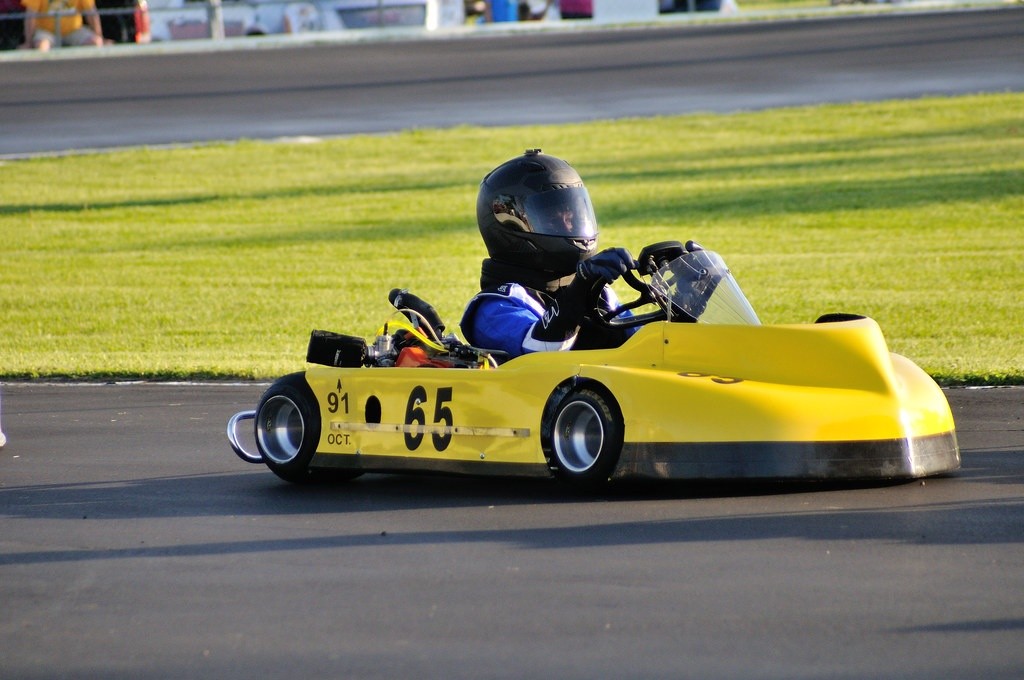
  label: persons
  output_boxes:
[0,0,741,49]
[459,149,664,364]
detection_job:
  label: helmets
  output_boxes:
[476,149,598,280]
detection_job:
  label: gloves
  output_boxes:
[569,248,640,298]
